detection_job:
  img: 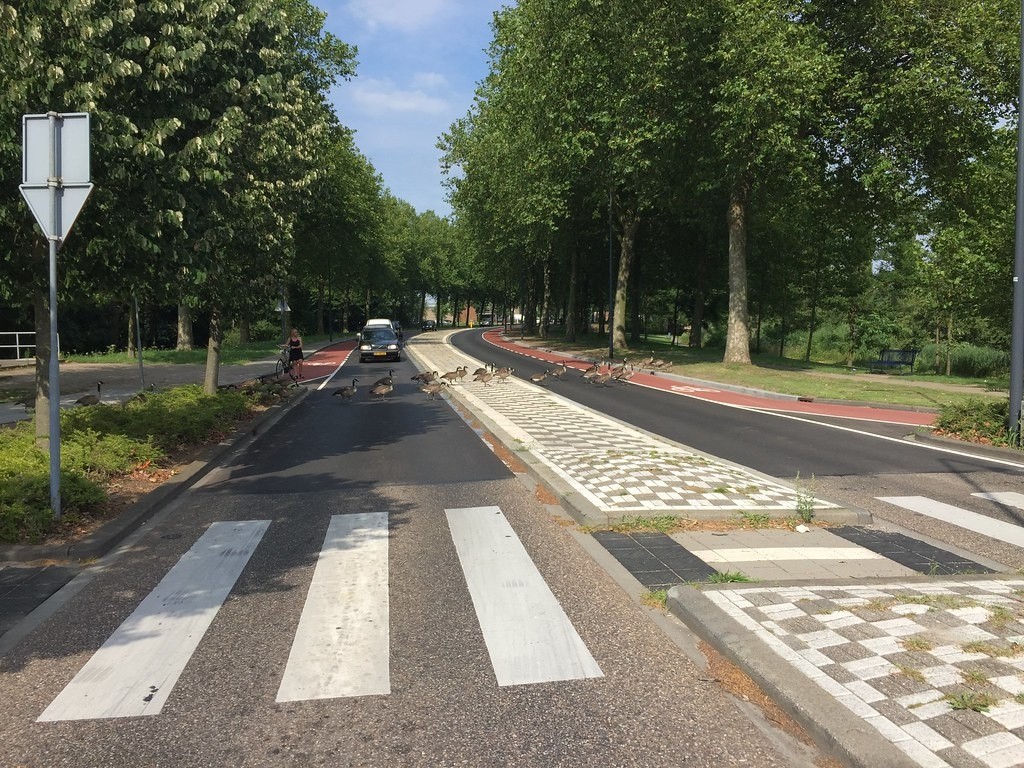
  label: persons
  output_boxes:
[286,328,305,378]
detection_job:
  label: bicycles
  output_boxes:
[276,345,296,380]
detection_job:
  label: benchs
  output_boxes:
[866,349,917,376]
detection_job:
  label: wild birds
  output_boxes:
[580,356,635,387]
[530,369,549,387]
[332,379,359,401]
[494,366,514,383]
[74,381,105,407]
[632,350,673,371]
[15,393,36,412]
[134,383,154,399]
[217,383,235,392]
[473,362,496,387]
[440,366,468,384]
[411,371,449,400]
[271,382,298,403]
[370,369,395,400]
[549,360,569,382]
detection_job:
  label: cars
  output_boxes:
[358,326,403,363]
[366,319,394,333]
[392,321,403,339]
[422,320,437,332]
[480,318,492,327]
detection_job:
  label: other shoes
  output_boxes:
[300,374,305,379]
[294,375,298,379]
